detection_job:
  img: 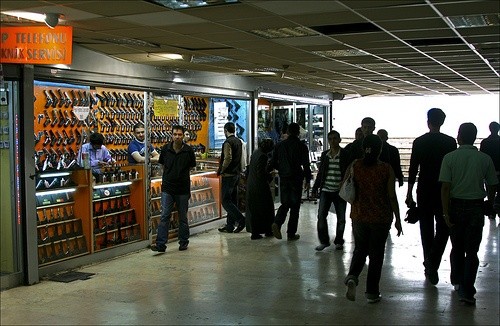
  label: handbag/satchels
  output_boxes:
[338,159,358,204]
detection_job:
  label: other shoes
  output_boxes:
[151,244,165,252]
[251,235,263,240]
[423,258,437,275]
[233,216,246,233]
[458,287,475,306]
[346,279,356,301]
[264,227,273,237]
[272,223,282,239]
[218,225,234,233]
[288,233,299,241]
[425,268,438,285]
[315,242,330,251]
[179,242,188,250]
[335,243,343,250]
[367,294,382,304]
[450,272,459,284]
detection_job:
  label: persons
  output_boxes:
[244,138,276,240]
[76,132,116,173]
[267,123,311,241]
[182,128,191,143]
[480,122,500,219]
[150,125,196,252]
[344,117,404,188]
[406,108,458,285]
[438,123,498,303]
[216,122,245,233]
[342,134,402,303]
[128,123,160,162]
[312,130,349,251]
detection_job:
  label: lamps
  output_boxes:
[182,53,194,63]
[44,13,59,29]
[276,72,285,80]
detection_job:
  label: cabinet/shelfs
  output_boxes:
[150,173,220,238]
[35,185,89,268]
[94,178,144,251]
